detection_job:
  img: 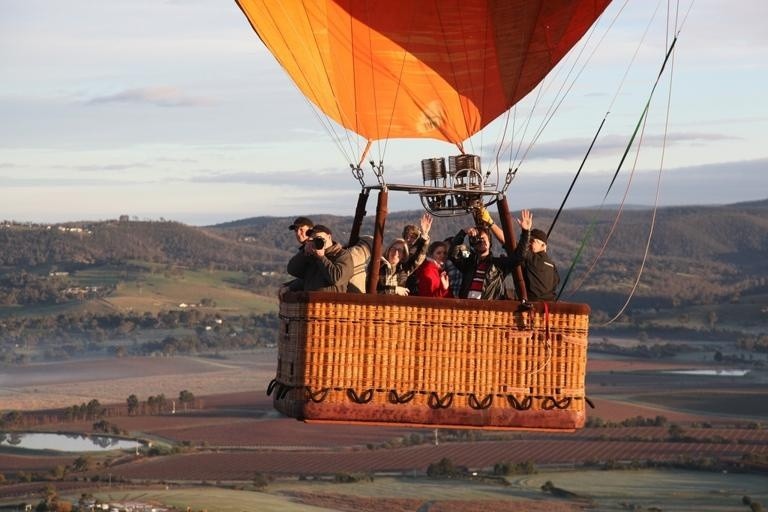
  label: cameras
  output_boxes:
[470,235,484,245]
[310,236,328,249]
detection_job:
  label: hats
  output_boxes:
[305,225,332,236]
[289,217,313,230]
[531,228,547,245]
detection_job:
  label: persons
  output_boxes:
[449,209,533,299]
[280,218,311,299]
[442,235,467,297]
[416,241,454,297]
[366,213,434,296]
[400,223,421,255]
[287,225,353,293]
[477,205,560,300]
[340,233,373,294]
